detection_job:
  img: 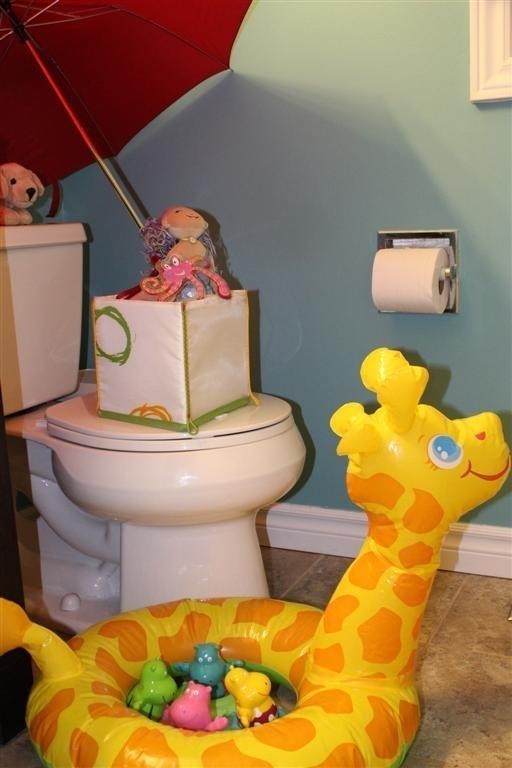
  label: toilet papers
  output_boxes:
[372,246,451,317]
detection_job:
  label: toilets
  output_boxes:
[1,221,307,637]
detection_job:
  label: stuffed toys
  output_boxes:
[117,209,231,301]
[0,163,45,224]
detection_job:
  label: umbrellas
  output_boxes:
[0,1,250,266]
